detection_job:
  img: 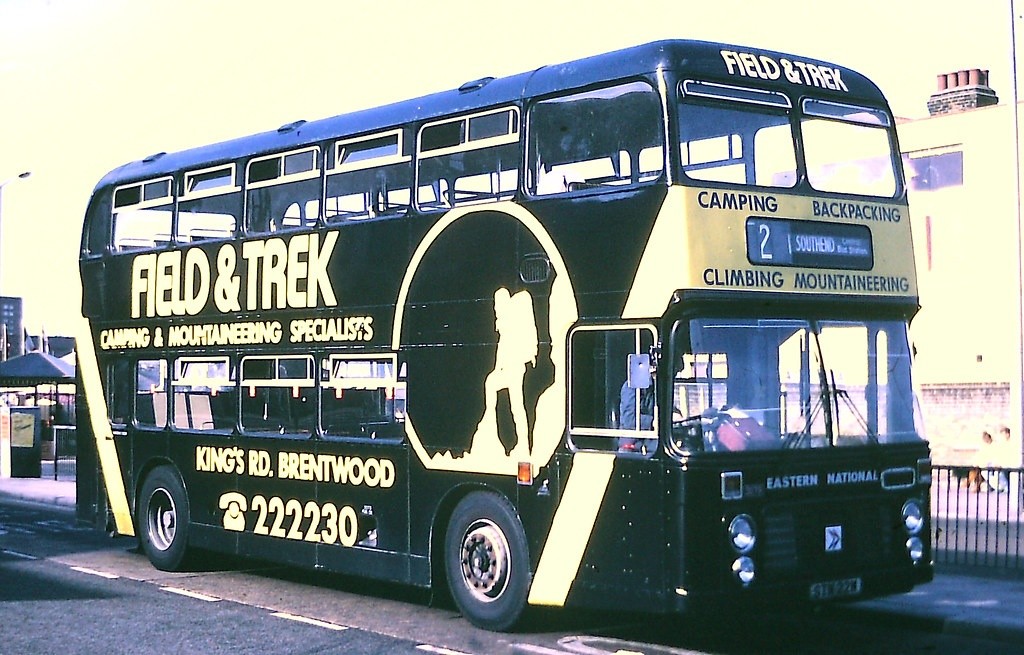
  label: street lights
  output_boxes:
[0,171,33,246]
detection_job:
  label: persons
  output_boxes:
[973,421,1011,492]
[614,349,686,456]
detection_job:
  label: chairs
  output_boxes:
[116,181,615,254]
[138,386,386,438]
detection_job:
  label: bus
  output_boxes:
[73,37,943,637]
